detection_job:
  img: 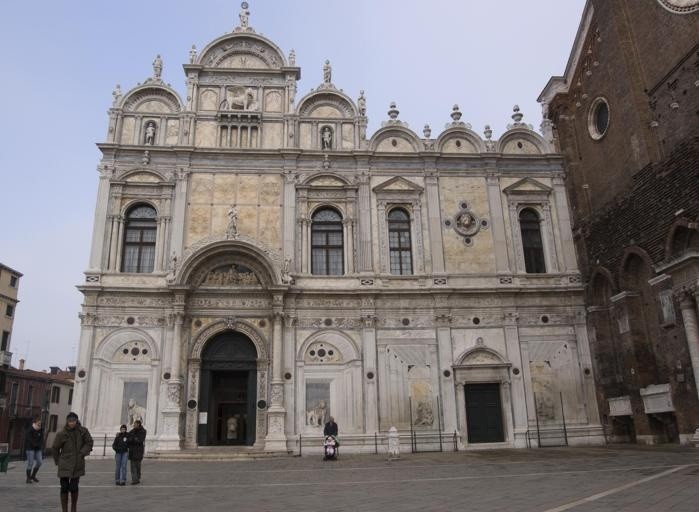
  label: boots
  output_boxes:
[26,469,31,483]
[32,468,39,482]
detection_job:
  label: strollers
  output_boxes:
[323,434,339,461]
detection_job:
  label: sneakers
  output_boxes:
[115,481,140,485]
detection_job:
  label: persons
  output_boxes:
[226,414,240,443]
[145,121,154,143]
[116,419,147,485]
[238,8,251,30]
[226,201,239,236]
[112,84,122,104]
[325,437,335,456]
[52,411,94,511]
[289,48,296,65]
[358,92,367,115]
[324,416,338,437]
[323,128,331,150]
[112,423,129,485]
[151,54,163,77]
[322,59,332,84]
[24,414,45,483]
[188,44,198,61]
